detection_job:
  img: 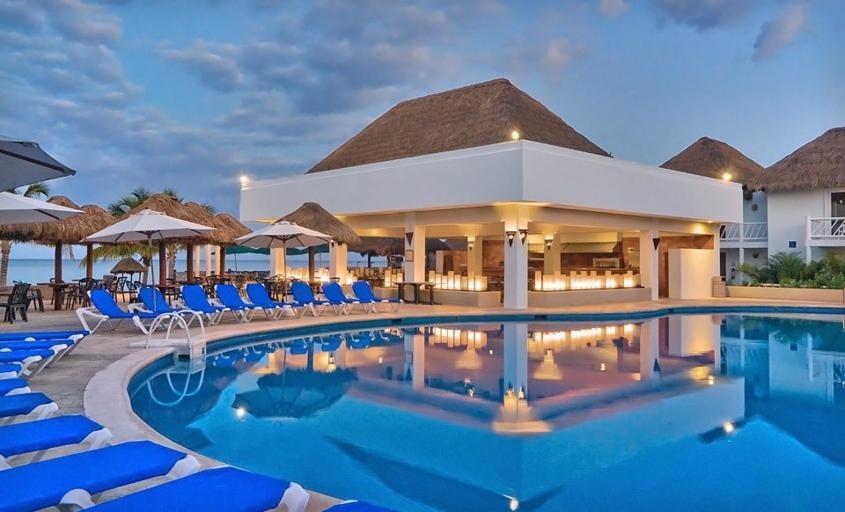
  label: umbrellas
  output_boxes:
[0,140,77,192]
[273,202,361,283]
[230,344,324,418]
[134,351,268,452]
[0,193,254,288]
[362,236,453,273]
[256,340,357,409]
[231,218,333,304]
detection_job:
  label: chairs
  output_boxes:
[2,392,58,425]
[0,412,112,465]
[1,349,57,380]
[2,377,32,395]
[1,339,76,368]
[0,438,201,511]
[1,330,91,356]
[321,497,395,511]
[59,463,310,511]
[0,270,433,335]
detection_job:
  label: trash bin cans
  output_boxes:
[103,275,117,291]
[713,276,727,298]
[713,315,726,325]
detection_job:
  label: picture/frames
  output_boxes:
[404,249,414,262]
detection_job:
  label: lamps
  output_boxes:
[517,227,530,244]
[544,238,554,250]
[505,379,516,398]
[653,356,662,374]
[505,229,517,246]
[466,239,475,252]
[518,383,527,402]
[405,230,415,247]
[652,237,661,251]
